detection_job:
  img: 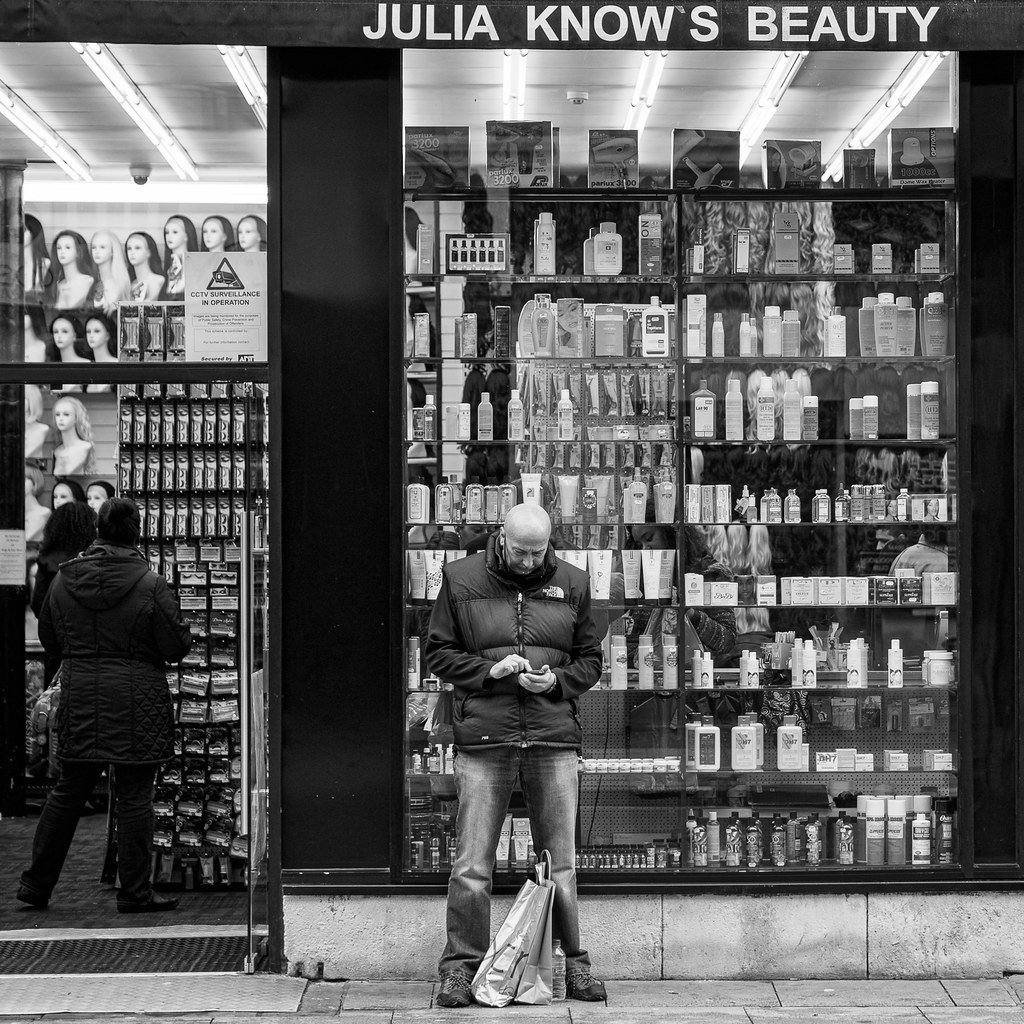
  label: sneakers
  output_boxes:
[565,972,606,1001]
[436,976,472,1007]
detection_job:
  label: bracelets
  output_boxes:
[545,673,556,693]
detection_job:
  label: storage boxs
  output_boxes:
[593,305,624,357]
[762,140,821,190]
[816,747,953,772]
[790,577,814,605]
[702,581,738,605]
[873,577,897,604]
[844,577,869,605]
[588,129,640,188]
[887,127,957,186]
[554,297,584,358]
[671,127,740,188]
[921,572,957,605]
[842,148,875,186]
[639,212,941,273]
[814,577,842,605]
[406,125,470,188]
[907,493,947,522]
[486,121,561,187]
[900,577,922,603]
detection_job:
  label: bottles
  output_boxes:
[408,201,958,870]
[551,938,566,1002]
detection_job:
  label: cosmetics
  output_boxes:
[406,212,959,870]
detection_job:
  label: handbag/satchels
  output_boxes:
[25,673,62,776]
[471,849,557,1007]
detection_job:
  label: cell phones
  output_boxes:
[528,670,545,675]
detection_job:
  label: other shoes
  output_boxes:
[17,882,49,907]
[116,888,179,912]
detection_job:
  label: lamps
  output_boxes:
[0,38,951,184]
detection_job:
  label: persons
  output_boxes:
[803,669,815,686]
[701,673,709,687]
[847,669,858,685]
[626,524,735,747]
[421,504,607,1009]
[881,524,949,669]
[889,669,901,686]
[924,499,940,521]
[886,500,898,520]
[748,671,758,685]
[23,384,116,541]
[559,301,582,348]
[737,577,756,604]
[23,214,268,362]
[14,498,192,915]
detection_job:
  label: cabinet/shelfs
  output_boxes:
[404,156,978,879]
[115,301,269,894]
